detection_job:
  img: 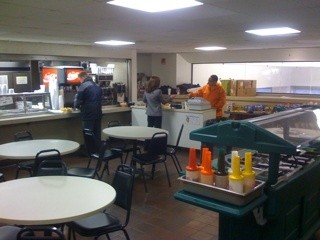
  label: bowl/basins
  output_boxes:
[0,83,14,94]
[16,101,43,110]
[34,85,45,92]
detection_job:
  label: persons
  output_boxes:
[187,75,226,122]
[74,72,103,157]
[143,76,165,150]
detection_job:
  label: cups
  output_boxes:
[49,76,64,109]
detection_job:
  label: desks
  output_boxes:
[130,106,216,151]
[0,175,117,240]
[0,139,81,176]
[102,126,168,168]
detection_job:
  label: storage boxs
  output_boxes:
[221,80,231,96]
[237,80,256,96]
[230,80,238,96]
[177,175,266,207]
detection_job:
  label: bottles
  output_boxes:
[216,152,229,189]
[229,151,243,194]
[199,148,213,185]
[242,152,256,193]
[186,148,200,182]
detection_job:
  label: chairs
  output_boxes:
[130,132,171,193]
[152,124,184,173]
[34,148,61,176]
[36,160,68,177]
[62,163,135,240]
[108,121,143,168]
[66,146,107,181]
[14,132,35,179]
[15,226,66,240]
[83,128,124,181]
